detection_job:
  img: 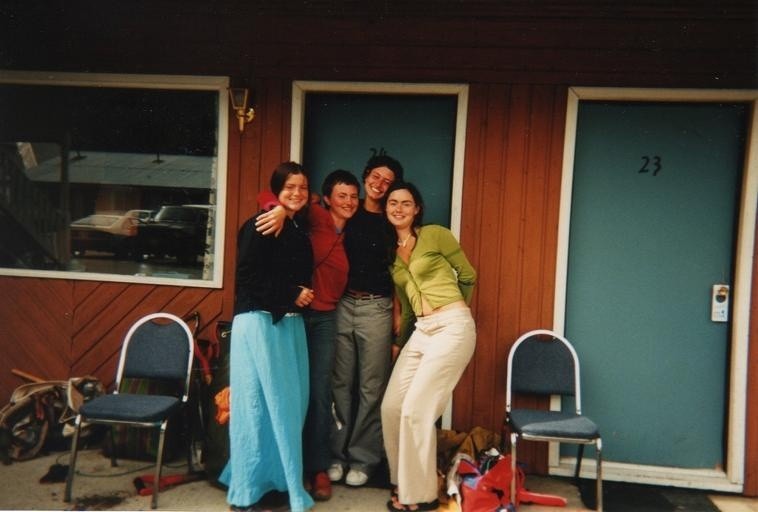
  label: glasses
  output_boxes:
[226,75,256,136]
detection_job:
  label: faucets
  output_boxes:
[390,486,398,496]
[386,496,441,512]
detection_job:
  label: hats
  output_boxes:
[71,205,208,265]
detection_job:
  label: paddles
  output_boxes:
[570,470,726,512]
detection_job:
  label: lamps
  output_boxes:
[62,311,197,510]
[500,328,605,510]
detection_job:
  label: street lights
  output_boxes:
[202,320,231,490]
[456,453,526,512]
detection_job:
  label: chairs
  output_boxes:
[326,463,349,481]
[312,471,332,502]
[345,464,369,486]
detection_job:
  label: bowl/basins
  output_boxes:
[396,232,412,248]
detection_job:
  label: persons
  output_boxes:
[308,155,403,488]
[252,168,362,502]
[379,177,477,512]
[216,160,315,512]
